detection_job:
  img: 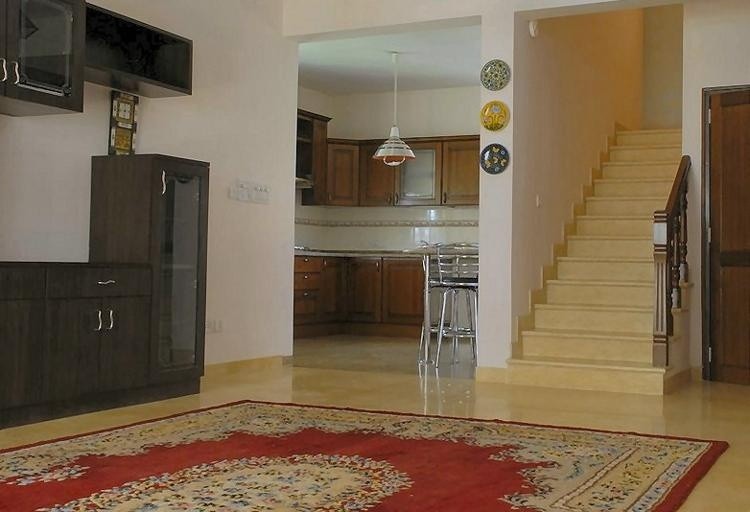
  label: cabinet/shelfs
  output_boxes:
[360,140,440,206]
[0,0,194,118]
[328,138,360,207]
[383,258,425,325]
[296,107,332,206]
[43,263,154,402]
[441,139,479,206]
[344,256,382,323]
[294,256,341,326]
[88,155,210,403]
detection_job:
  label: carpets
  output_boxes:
[0,398,729,512]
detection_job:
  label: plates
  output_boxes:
[480,101,510,132]
[480,143,509,173]
[480,59,511,91]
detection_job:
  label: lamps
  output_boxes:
[372,52,416,166]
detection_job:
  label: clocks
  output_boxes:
[107,89,139,155]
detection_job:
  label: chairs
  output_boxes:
[434,256,479,368]
[417,253,479,363]
